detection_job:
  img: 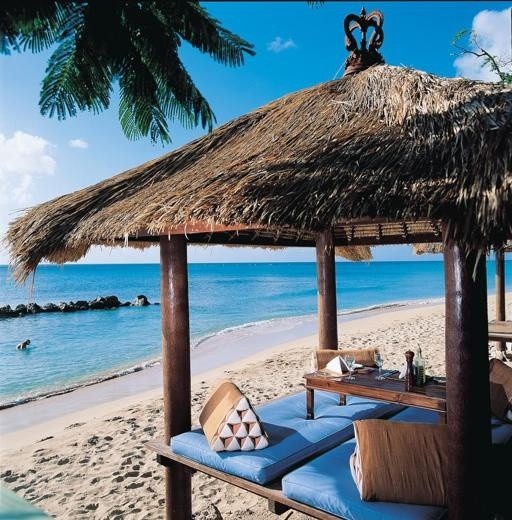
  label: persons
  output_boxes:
[16,340,30,350]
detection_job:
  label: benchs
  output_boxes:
[279,404,512,520]
[169,387,406,487]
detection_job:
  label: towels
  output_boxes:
[322,353,351,375]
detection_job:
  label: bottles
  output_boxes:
[414,348,427,386]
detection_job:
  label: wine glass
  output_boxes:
[372,349,387,382]
[345,353,357,381]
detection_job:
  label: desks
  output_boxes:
[299,358,449,426]
